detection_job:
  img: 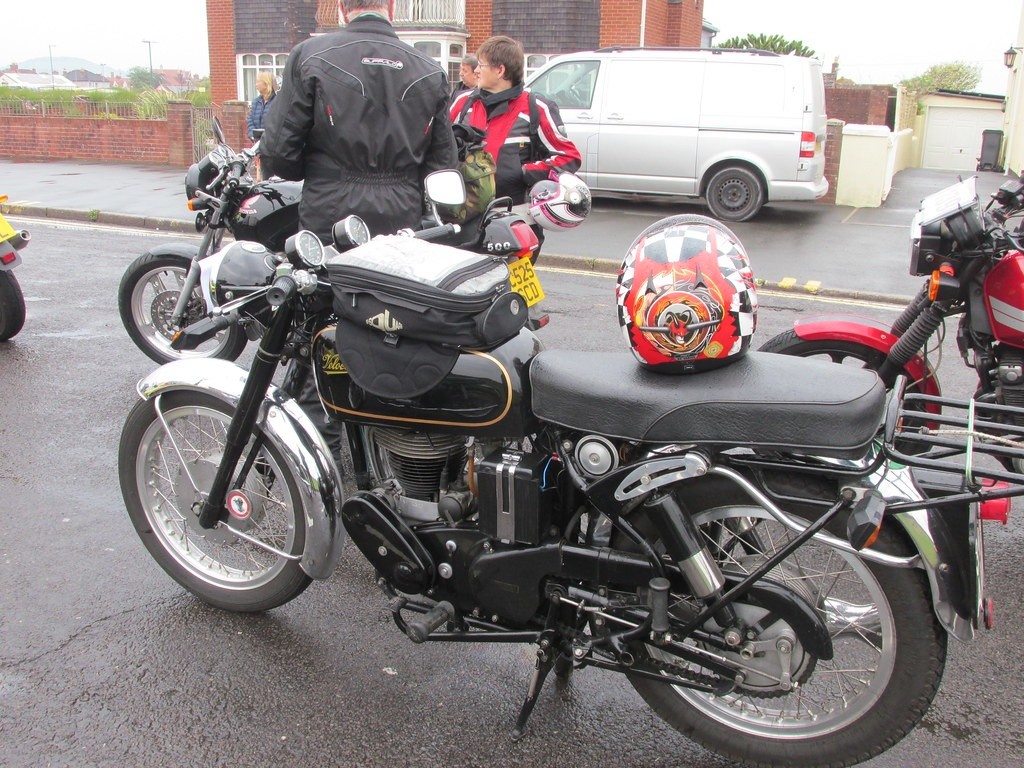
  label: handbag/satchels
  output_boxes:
[435,123,497,226]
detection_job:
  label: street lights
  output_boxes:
[48,44,57,91]
[142,39,160,89]
[100,63,107,92]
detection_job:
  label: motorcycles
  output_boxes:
[115,168,1024,768]
[0,193,32,342]
[752,168,1023,484]
[114,115,551,365]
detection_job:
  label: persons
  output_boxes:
[437,35,582,266]
[246,70,280,181]
[257,0,458,489]
[450,52,478,105]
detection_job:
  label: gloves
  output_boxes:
[520,165,548,188]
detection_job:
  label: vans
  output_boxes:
[520,44,831,224]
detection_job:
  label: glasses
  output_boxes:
[477,60,498,69]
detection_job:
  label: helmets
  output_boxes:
[615,215,760,376]
[528,170,591,232]
